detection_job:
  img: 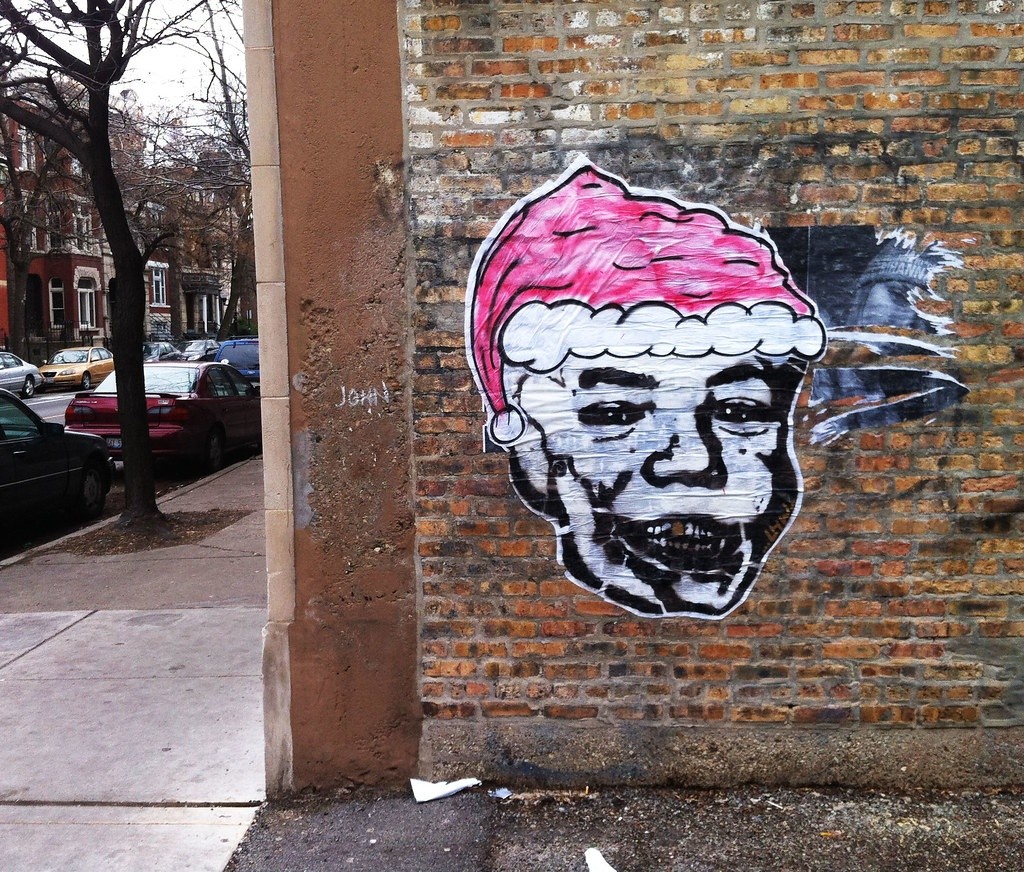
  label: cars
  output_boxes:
[1,388,119,546]
[141,341,182,364]
[1,352,44,396]
[65,361,261,478]
[37,346,115,388]
[175,340,221,363]
[212,334,260,393]
